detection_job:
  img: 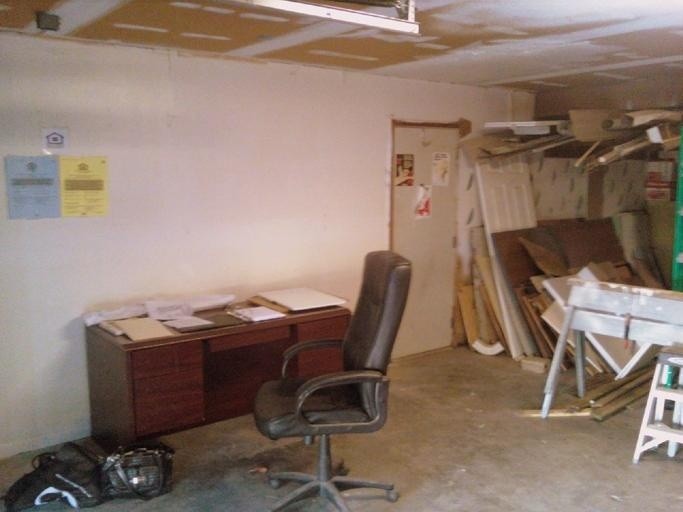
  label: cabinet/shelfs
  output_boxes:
[86,285,352,445]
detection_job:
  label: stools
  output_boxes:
[632,351,683,465]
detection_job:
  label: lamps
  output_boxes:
[239,0,422,36]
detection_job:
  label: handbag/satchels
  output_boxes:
[5,429,120,511]
[100,446,174,497]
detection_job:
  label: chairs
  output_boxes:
[252,251,411,511]
